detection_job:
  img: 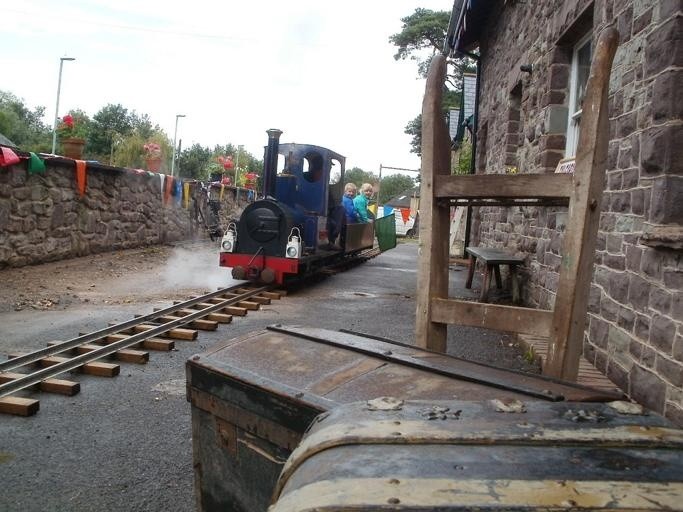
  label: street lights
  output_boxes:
[170,114,186,176]
[52,56,75,155]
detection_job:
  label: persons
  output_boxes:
[353,183,374,223]
[342,183,365,224]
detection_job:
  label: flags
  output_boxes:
[374,213,396,251]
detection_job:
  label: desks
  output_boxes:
[465,247,525,305]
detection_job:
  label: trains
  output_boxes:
[234,145,244,187]
[218,129,375,285]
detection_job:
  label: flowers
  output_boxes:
[55,116,85,138]
[143,143,160,160]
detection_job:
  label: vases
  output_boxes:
[146,159,161,173]
[60,139,86,160]
